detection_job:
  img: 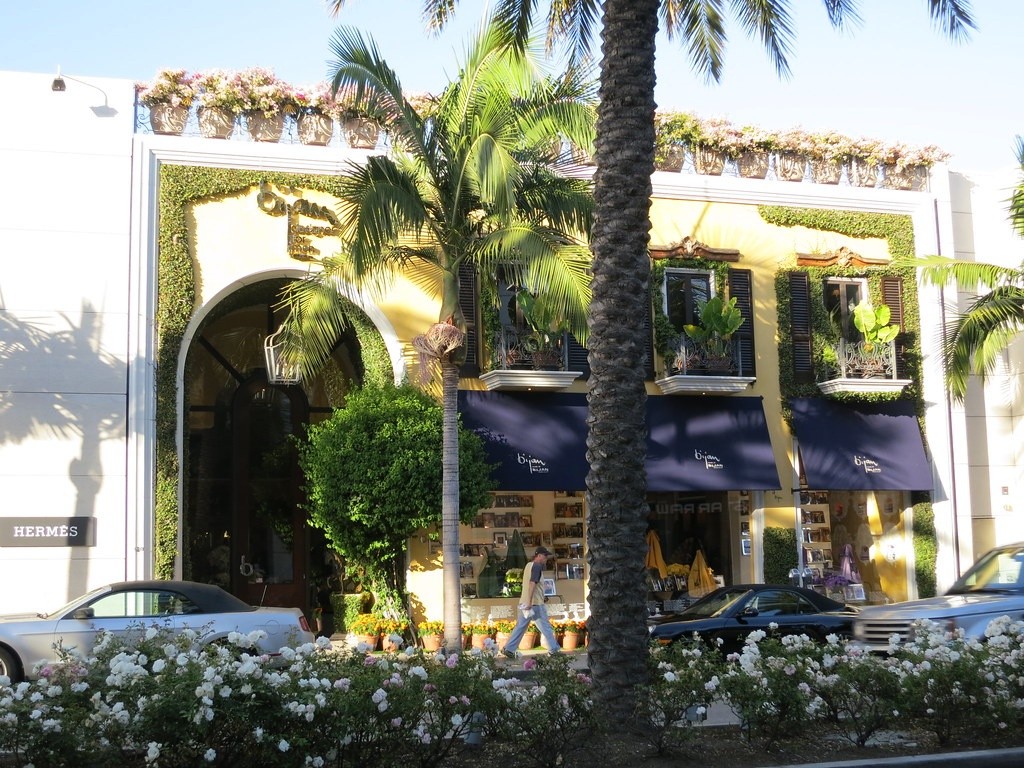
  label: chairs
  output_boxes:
[157,594,171,613]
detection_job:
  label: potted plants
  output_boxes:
[683,297,745,374]
[854,299,901,374]
[517,289,570,371]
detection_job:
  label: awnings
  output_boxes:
[456,388,934,493]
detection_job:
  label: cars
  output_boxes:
[853,540,1024,658]
[0,578,317,688]
[645,583,861,662]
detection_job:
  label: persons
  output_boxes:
[465,499,583,593]
[501,546,566,658]
[804,493,826,524]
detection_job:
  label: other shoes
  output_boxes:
[500,649,518,658]
[548,652,566,658]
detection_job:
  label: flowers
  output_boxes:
[461,619,587,636]
[417,620,444,639]
[135,65,949,172]
[350,614,408,636]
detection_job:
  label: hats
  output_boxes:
[535,547,553,556]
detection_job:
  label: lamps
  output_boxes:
[51,64,107,98]
[263,321,306,385]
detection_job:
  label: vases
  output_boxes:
[358,634,377,651]
[379,633,402,652]
[421,633,444,652]
[297,115,334,146]
[389,125,415,152]
[808,160,843,185]
[690,149,725,175]
[472,631,588,651]
[246,109,284,142]
[569,139,596,166]
[539,135,563,163]
[655,145,684,173]
[773,153,807,182]
[738,152,770,179]
[150,103,190,136]
[342,118,382,149]
[846,162,879,187]
[882,165,914,190]
[461,634,467,649]
[196,106,236,140]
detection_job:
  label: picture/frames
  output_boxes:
[742,538,751,555]
[494,532,507,548]
[506,512,520,527]
[810,511,825,523]
[816,492,829,504]
[471,515,483,528]
[805,547,866,601]
[521,531,551,547]
[496,495,534,508]
[808,530,820,543]
[464,543,493,556]
[741,522,750,536]
[482,513,496,528]
[459,562,569,597]
[519,515,532,527]
[495,515,506,527]
[819,527,831,542]
[552,491,584,559]
[801,508,806,524]
[740,500,749,515]
